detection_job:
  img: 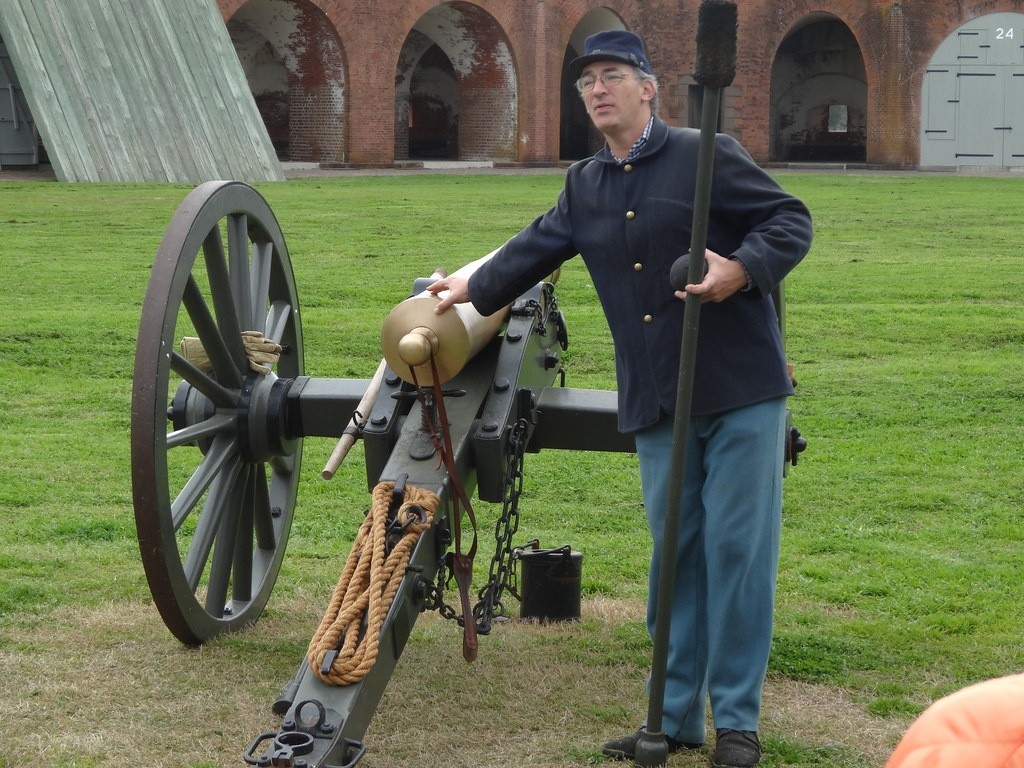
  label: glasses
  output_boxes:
[574,71,641,93]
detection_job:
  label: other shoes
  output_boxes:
[713,728,764,768]
[602,726,704,761]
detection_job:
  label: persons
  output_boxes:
[426,30,813,767]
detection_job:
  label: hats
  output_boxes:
[569,30,651,74]
